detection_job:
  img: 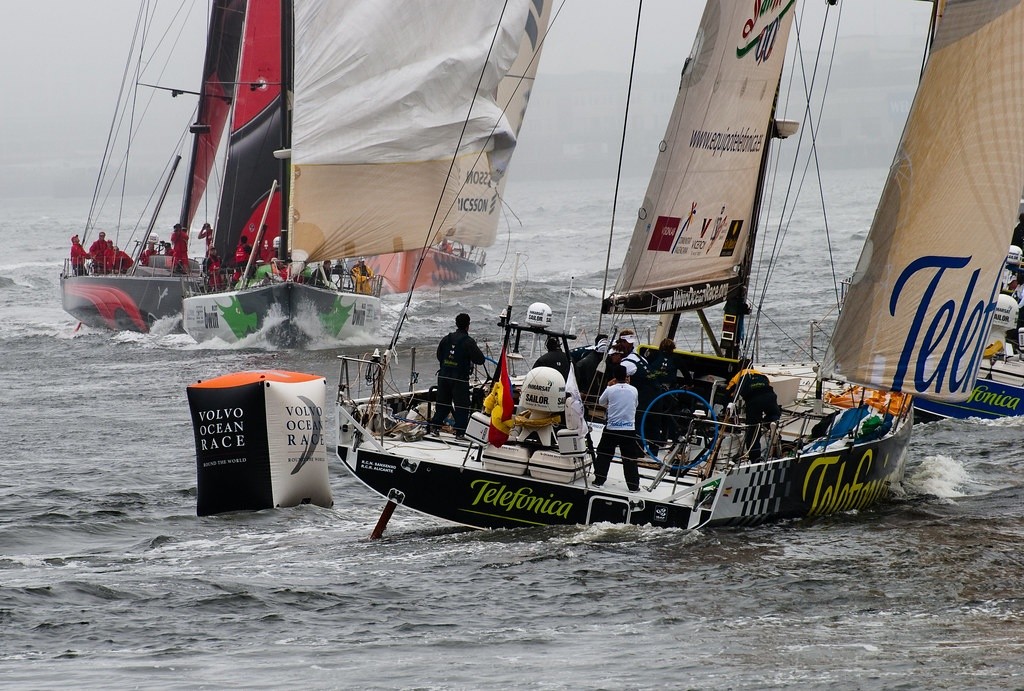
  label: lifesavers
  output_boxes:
[515,409,561,428]
[983,341,1003,359]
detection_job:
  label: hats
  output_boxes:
[607,348,625,356]
[271,258,278,263]
[357,256,366,263]
[106,240,112,244]
[174,223,182,229]
[71,235,78,242]
[165,243,171,248]
[204,223,212,230]
[595,339,609,348]
[619,335,639,344]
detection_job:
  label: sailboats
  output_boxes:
[60,0,292,334]
[335,1,1024,536]
[183,0,532,352]
[338,0,552,297]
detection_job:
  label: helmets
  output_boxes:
[546,338,562,347]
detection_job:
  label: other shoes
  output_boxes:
[629,487,640,493]
[644,453,657,460]
[592,481,604,488]
[430,429,440,438]
[454,435,471,443]
[763,421,770,432]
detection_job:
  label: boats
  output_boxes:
[840,244,1024,424]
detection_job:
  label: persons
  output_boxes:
[1006,276,1024,339]
[71,222,214,275]
[727,358,782,464]
[439,237,452,254]
[431,312,485,438]
[592,364,640,492]
[585,330,676,458]
[206,236,373,294]
